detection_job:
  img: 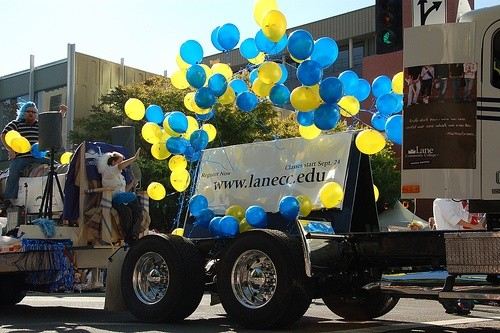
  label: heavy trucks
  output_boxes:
[0,3,500,333]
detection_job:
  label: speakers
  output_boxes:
[112,126,135,157]
[37,111,62,151]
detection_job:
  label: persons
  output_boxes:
[97,147,141,246]
[0,102,68,207]
[406,63,476,107]
[432,198,484,230]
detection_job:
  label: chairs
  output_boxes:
[85,141,150,246]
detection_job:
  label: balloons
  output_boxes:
[31,142,47,158]
[60,151,73,164]
[5,130,21,147]
[125,2,404,236]
[11,137,32,153]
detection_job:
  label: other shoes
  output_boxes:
[445,303,471,315]
[0,199,11,216]
[406,103,412,108]
[422,97,429,104]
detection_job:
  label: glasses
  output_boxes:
[25,110,37,114]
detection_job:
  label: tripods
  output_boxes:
[38,151,65,218]
